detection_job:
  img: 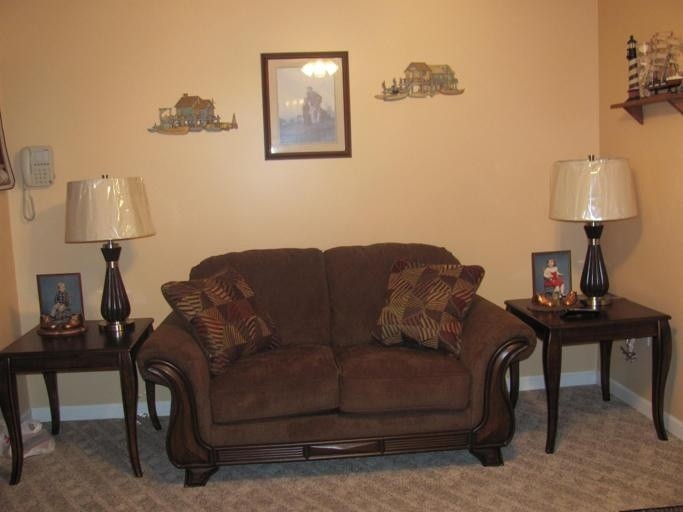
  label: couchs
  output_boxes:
[138,243,536,487]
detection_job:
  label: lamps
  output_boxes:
[548,152,638,305]
[64,171,154,334]
[300,59,338,79]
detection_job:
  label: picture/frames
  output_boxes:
[260,50,353,161]
[34,271,86,327]
[531,250,573,302]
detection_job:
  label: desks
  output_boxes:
[0,317,162,484]
[507,289,671,452]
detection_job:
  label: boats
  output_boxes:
[643,30,683,93]
[146,118,237,137]
[373,80,466,103]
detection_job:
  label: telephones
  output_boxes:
[20,145,54,187]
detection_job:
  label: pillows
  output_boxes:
[375,259,484,362]
[164,263,279,377]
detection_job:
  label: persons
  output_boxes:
[543,257,566,300]
[50,281,72,319]
